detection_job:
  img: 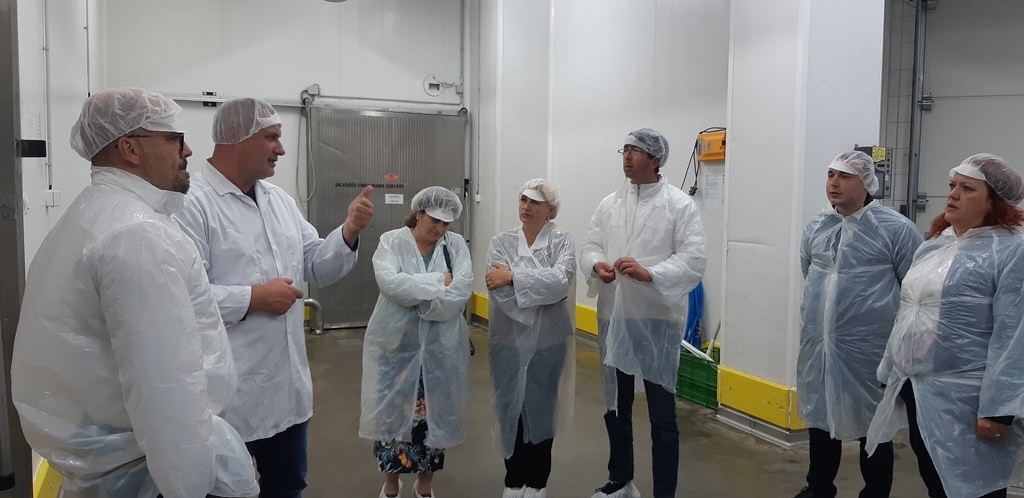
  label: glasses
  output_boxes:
[420,214,449,226]
[115,133,184,152]
[618,147,648,156]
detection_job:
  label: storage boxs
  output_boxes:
[675,346,720,411]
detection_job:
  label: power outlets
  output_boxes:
[476,195,480,202]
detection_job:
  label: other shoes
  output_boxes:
[794,481,837,498]
[595,481,626,493]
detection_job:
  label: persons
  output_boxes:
[865,153,1024,498]
[795,151,924,498]
[359,186,475,498]
[11,87,259,498]
[169,98,375,498]
[485,178,577,498]
[579,128,707,498]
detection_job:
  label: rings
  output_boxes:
[487,281,491,285]
[993,434,1000,438]
